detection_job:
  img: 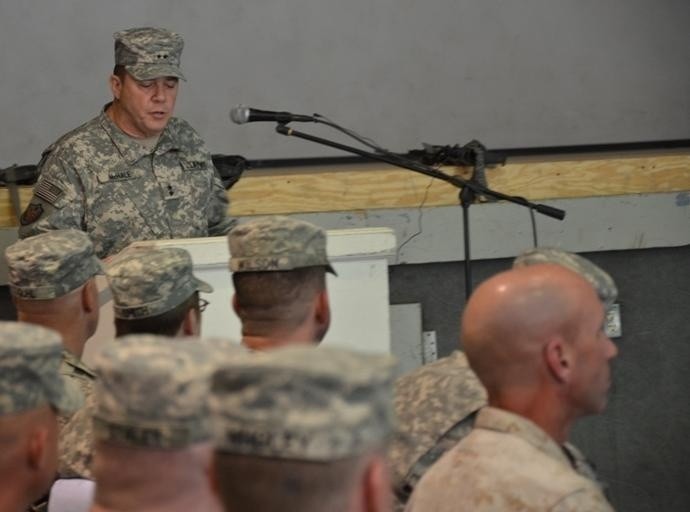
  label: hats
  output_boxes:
[113,26,188,82]
[227,215,338,278]
[92,333,251,452]
[202,344,418,461]
[106,245,213,320]
[4,227,106,300]
[0,320,85,414]
[514,247,619,319]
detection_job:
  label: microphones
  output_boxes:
[229,106,312,124]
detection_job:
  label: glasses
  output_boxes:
[193,297,210,313]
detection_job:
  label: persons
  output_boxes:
[5,228,106,478]
[20,28,235,242]
[228,215,338,349]
[105,241,214,336]
[211,345,399,510]
[92,336,214,510]
[0,321,85,511]
